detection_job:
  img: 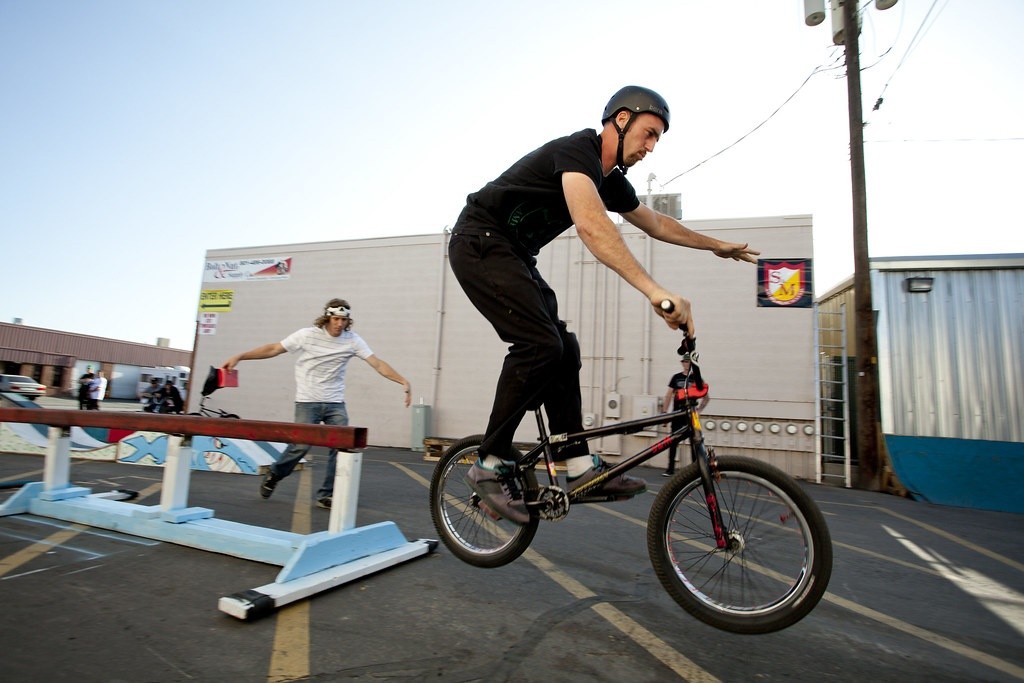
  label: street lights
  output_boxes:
[804,0,896,489]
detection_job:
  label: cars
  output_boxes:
[0,374,47,401]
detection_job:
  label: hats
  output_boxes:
[87,364,92,368]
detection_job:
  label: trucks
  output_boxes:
[137,364,190,402]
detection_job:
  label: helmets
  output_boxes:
[601,85,670,133]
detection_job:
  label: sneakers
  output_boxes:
[462,456,530,526]
[316,495,331,509]
[260,469,278,498]
[566,452,648,497]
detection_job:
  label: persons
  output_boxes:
[86,370,107,410]
[221,298,411,510]
[140,377,184,414]
[448,86,761,525]
[76,365,96,409]
[662,354,709,477]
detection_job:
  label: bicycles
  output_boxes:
[186,394,242,421]
[428,299,834,635]
